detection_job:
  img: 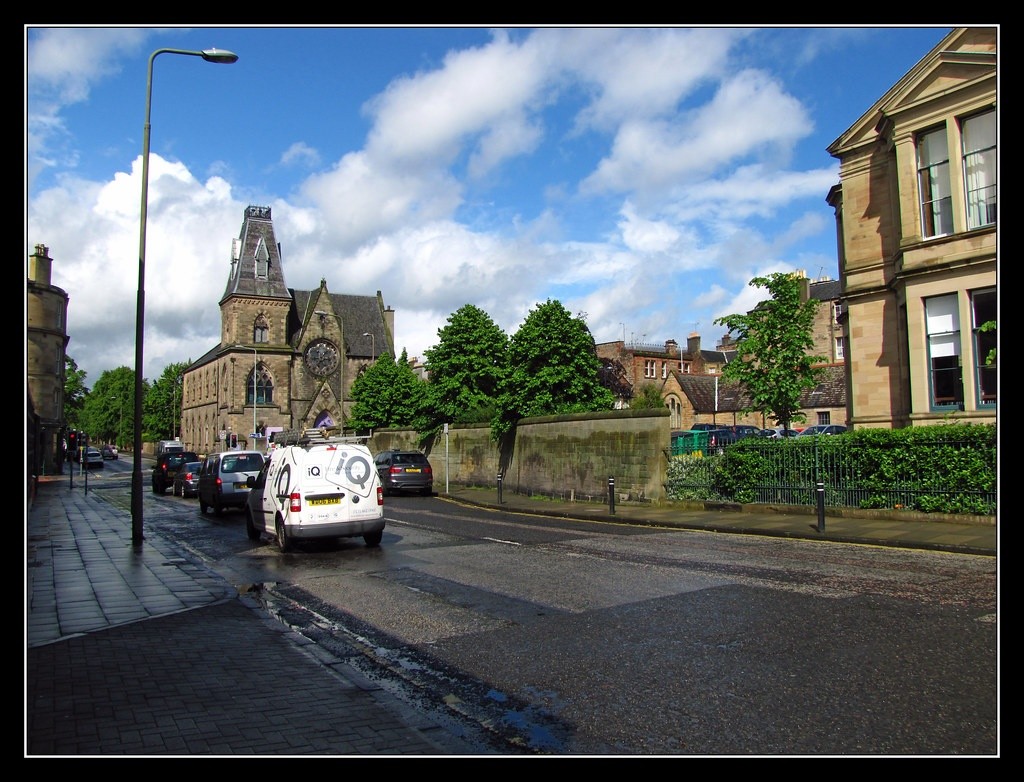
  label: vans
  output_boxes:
[157,440,184,457]
[243,424,387,553]
[195,450,267,515]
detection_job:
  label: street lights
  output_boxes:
[236,344,257,451]
[126,44,239,545]
[363,332,375,366]
[314,310,345,437]
[158,377,175,440]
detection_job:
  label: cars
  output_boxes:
[101,444,118,460]
[84,446,98,454]
[795,428,816,435]
[172,460,206,499]
[85,452,104,469]
[757,428,799,440]
[691,422,762,456]
[794,424,847,437]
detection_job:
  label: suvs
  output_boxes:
[372,449,434,497]
[151,451,201,494]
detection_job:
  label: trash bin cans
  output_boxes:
[671,430,709,456]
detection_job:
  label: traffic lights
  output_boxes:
[69,432,77,450]
[230,434,238,448]
[225,434,231,448]
